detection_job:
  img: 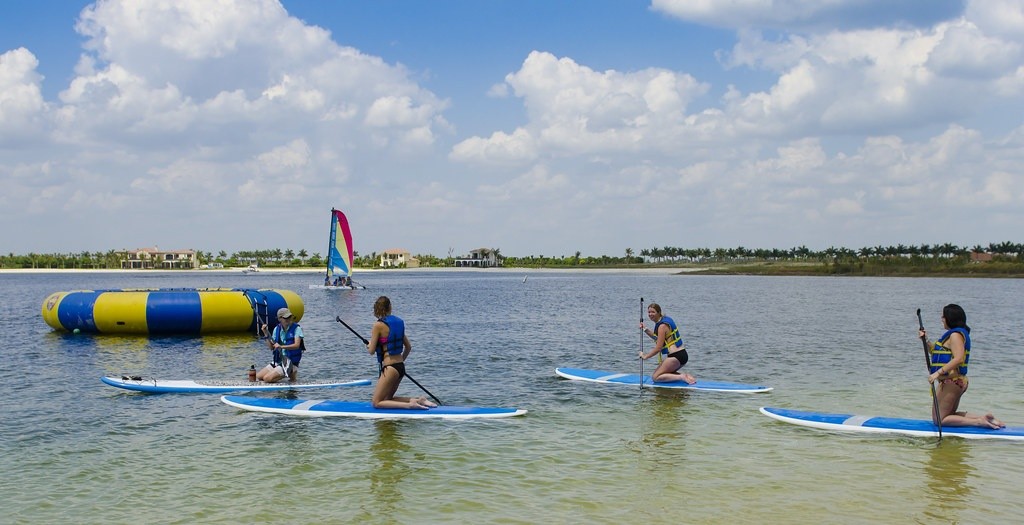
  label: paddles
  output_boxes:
[242,288,294,377]
[916,308,943,440]
[335,315,444,406]
[639,297,645,390]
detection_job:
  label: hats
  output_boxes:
[277,308,292,319]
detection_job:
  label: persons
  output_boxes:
[256,308,304,382]
[919,304,1006,430]
[639,303,697,385]
[325,276,352,286]
[364,296,437,409]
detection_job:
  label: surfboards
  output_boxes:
[220,394,528,419]
[759,405,1024,443]
[554,366,774,394]
[100,377,372,394]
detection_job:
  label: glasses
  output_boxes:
[283,315,291,319]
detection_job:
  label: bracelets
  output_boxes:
[937,370,940,374]
[941,367,945,373]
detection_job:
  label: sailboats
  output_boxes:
[309,206,366,291]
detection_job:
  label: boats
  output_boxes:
[41,286,305,334]
